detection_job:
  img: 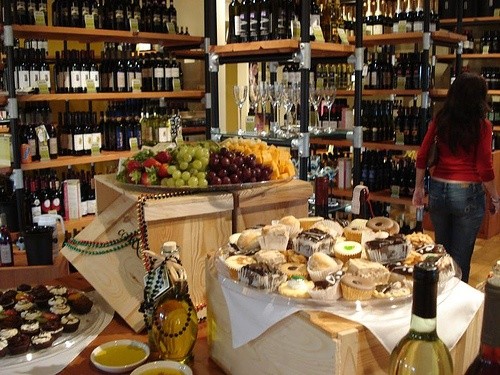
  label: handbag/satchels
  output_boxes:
[425,115,443,169]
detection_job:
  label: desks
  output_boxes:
[0,272,227,375]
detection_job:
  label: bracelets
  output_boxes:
[491,195,500,204]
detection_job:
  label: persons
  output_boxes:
[250,63,260,102]
[412,72,500,284]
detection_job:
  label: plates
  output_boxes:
[130,360,193,375]
[206,223,462,318]
[110,169,292,190]
[90,339,150,373]
[0,286,106,372]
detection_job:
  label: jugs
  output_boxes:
[32,212,66,265]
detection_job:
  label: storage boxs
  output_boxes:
[93,172,485,375]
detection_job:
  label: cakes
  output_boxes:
[224,216,455,302]
[0,282,94,357]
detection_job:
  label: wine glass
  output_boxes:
[233,81,341,136]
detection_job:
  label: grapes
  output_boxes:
[206,147,273,184]
[160,144,217,187]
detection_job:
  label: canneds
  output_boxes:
[63,180,82,220]
[341,108,354,129]
[21,143,32,164]
[336,157,351,188]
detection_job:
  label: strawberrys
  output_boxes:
[116,148,176,185]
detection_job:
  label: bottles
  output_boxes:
[4,164,103,222]
[0,211,16,268]
[147,241,201,362]
[251,61,355,92]
[0,0,190,169]
[351,39,500,203]
[225,0,499,56]
[389,262,453,375]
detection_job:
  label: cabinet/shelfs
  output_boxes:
[0,0,500,288]
